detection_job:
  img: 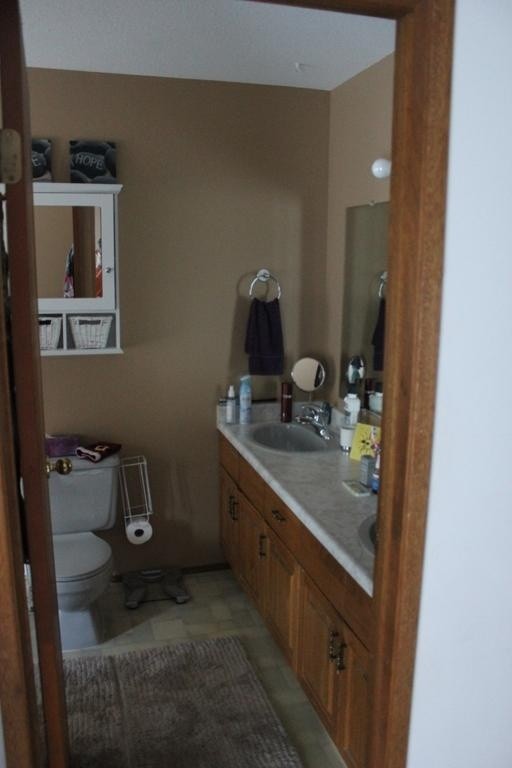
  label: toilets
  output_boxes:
[44,449,122,652]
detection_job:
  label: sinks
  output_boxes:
[248,416,332,456]
[358,512,379,560]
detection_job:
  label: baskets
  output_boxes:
[35,316,63,351]
[68,316,114,350]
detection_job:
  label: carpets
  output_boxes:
[31,635,306,768]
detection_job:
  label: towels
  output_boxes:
[244,296,285,376]
[370,297,386,373]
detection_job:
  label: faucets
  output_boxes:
[294,401,336,442]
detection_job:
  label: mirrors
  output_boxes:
[31,192,116,314]
[334,201,390,419]
